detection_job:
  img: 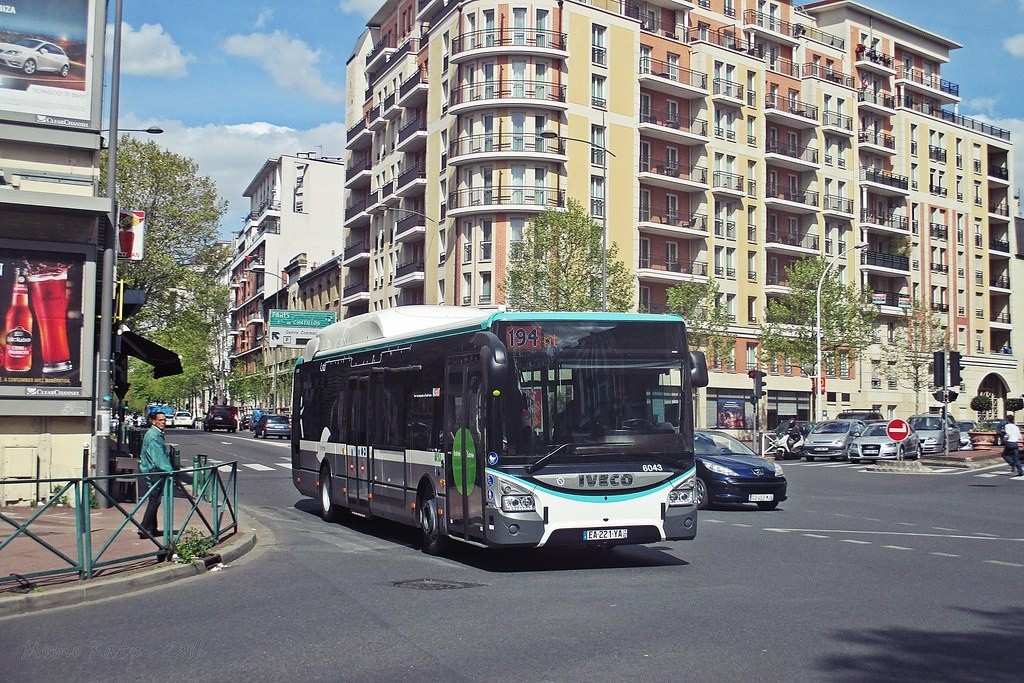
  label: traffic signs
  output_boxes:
[269,309,338,347]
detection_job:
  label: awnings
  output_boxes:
[122,331,184,379]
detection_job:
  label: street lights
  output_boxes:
[817,241,869,422]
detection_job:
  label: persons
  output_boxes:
[784,418,801,450]
[1001,415,1024,476]
[137,412,174,539]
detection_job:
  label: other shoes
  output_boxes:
[148,530,163,537]
[137,529,148,539]
[1011,463,1017,471]
[1016,472,1024,476]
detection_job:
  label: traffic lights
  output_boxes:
[750,396,758,404]
[950,351,965,386]
[125,401,128,410]
[120,279,146,321]
[748,370,756,378]
[754,370,766,399]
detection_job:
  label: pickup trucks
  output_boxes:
[172,413,193,429]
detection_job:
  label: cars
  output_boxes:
[239,414,292,440]
[805,420,922,463]
[956,419,1009,451]
[693,428,788,511]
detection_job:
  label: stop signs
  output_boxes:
[886,419,910,442]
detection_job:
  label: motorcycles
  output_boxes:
[775,422,804,460]
[250,410,264,431]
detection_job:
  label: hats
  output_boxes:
[788,418,795,427]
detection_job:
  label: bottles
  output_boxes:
[5,268,33,372]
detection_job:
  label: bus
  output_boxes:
[291,305,708,555]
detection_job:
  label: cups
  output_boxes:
[27,272,73,373]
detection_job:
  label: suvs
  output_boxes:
[836,409,961,455]
[165,415,174,428]
[203,405,238,432]
